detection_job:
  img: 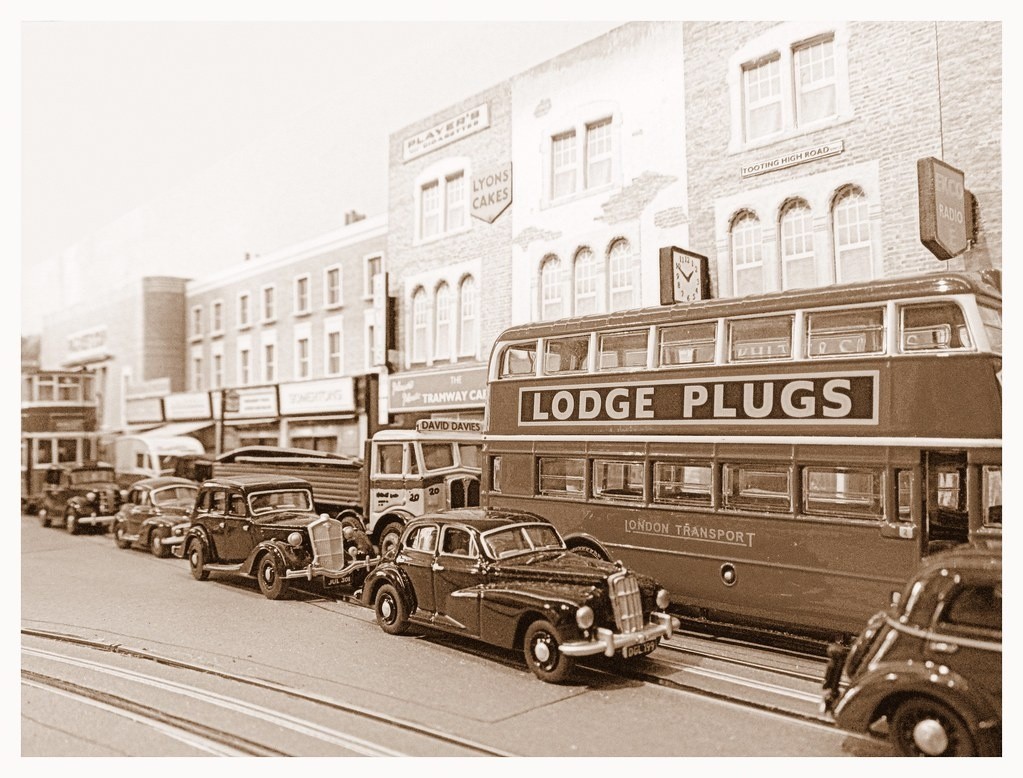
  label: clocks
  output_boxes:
[660,246,709,308]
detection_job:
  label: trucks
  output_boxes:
[215,418,483,562]
[111,434,203,494]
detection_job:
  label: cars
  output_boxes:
[38,464,123,535]
[821,554,1002,757]
[116,479,203,559]
[171,475,383,603]
[361,507,681,685]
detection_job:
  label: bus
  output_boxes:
[479,270,1003,637]
[21,403,103,514]
